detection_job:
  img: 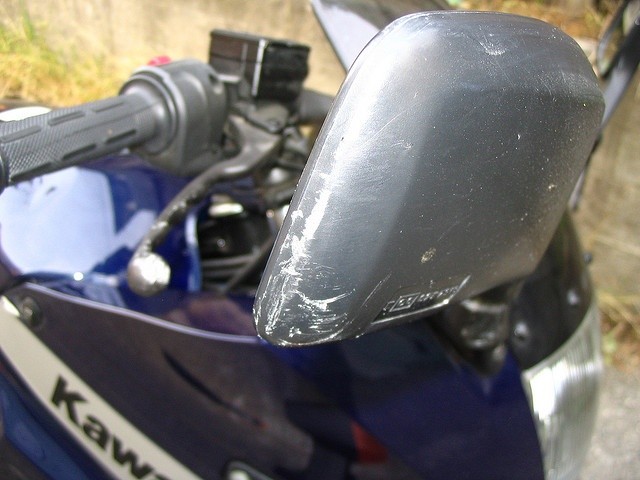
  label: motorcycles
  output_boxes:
[1,0,640,479]
